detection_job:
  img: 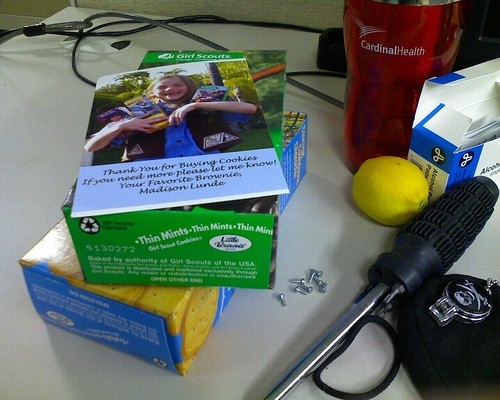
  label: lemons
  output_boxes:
[351,155,430,227]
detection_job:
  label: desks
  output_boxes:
[0,6,500,400]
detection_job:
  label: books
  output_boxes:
[70,57,291,218]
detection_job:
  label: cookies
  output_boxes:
[19,217,219,360]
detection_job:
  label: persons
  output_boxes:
[84,74,258,164]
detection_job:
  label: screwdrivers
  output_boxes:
[264,175,500,400]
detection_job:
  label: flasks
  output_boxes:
[340,0,466,176]
